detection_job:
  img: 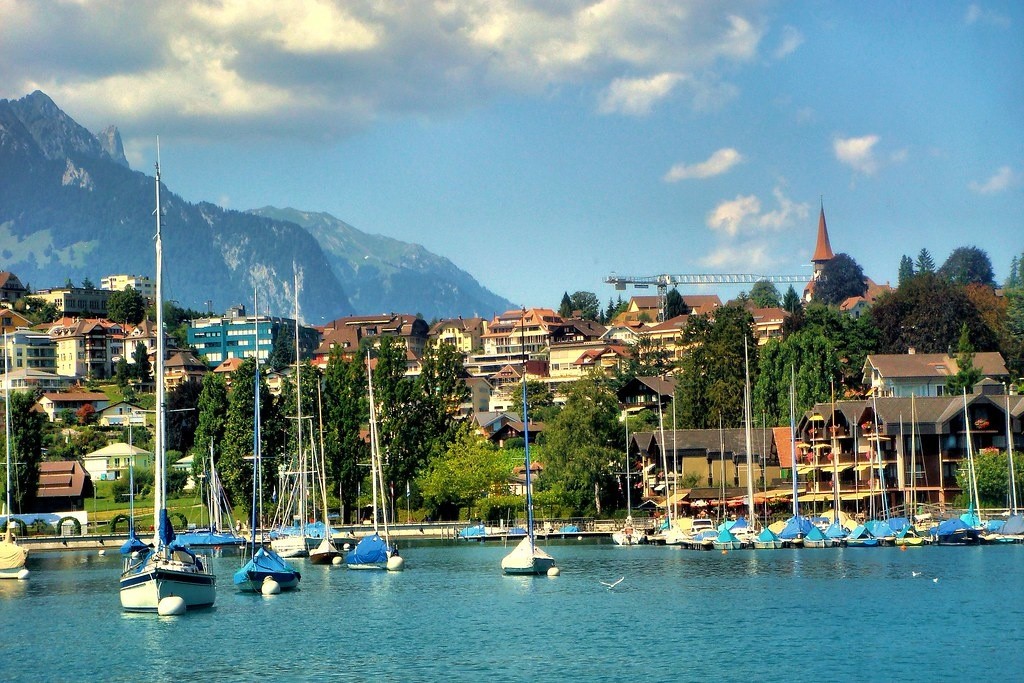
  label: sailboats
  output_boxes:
[610,332,1024,546]
[346,348,399,569]
[172,435,248,554]
[234,286,302,593]
[308,374,343,564]
[501,304,558,576]
[118,411,151,560]
[117,137,219,617]
[0,327,29,579]
[264,274,356,559]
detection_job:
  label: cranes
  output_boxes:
[601,272,826,324]
